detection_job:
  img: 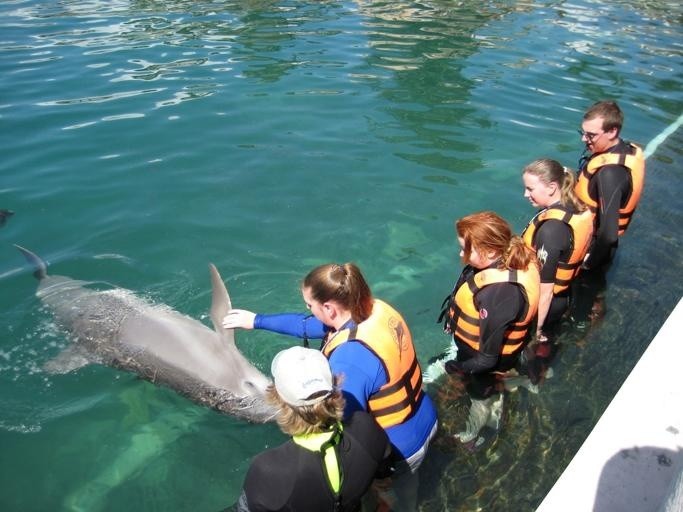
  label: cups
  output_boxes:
[577,129,610,139]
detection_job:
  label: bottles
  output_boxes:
[270,344,333,407]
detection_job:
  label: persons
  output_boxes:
[518,157,596,394]
[571,101,646,325]
[222,259,439,512]
[221,344,397,511]
[440,211,541,447]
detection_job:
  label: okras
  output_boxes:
[13,244,291,424]
